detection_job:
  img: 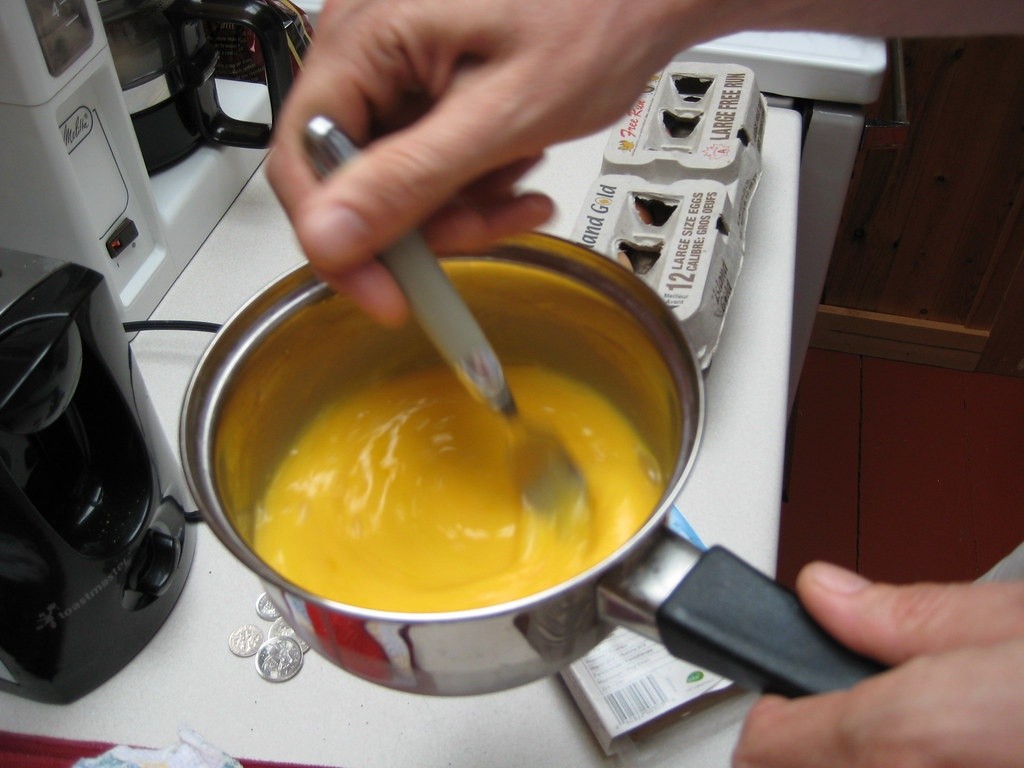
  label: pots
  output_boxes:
[179,228,895,696]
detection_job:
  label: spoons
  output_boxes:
[302,115,584,521]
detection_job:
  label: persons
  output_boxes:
[261,1,1024,768]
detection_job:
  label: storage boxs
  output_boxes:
[561,506,739,757]
[573,61,769,375]
